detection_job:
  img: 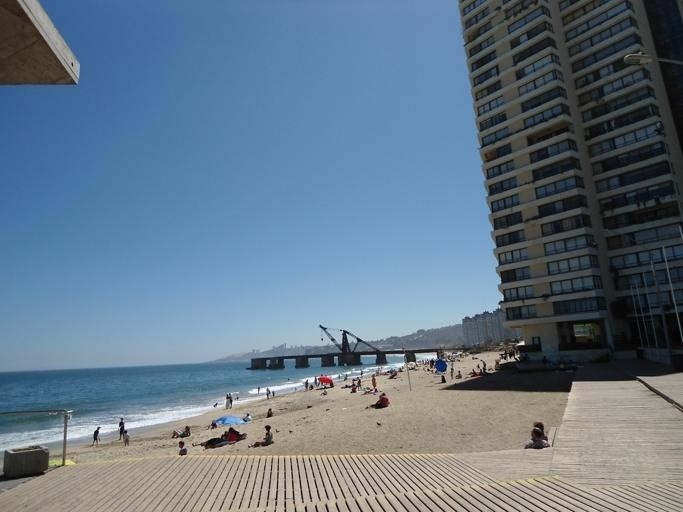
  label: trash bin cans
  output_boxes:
[636,349,644,360]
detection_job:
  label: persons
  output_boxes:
[92,426,101,448]
[533,422,548,443]
[525,427,550,448]
[123,431,131,446]
[172,426,191,438]
[179,441,187,456]
[304,349,547,397]
[117,417,125,440]
[367,392,390,409]
[192,386,274,451]
